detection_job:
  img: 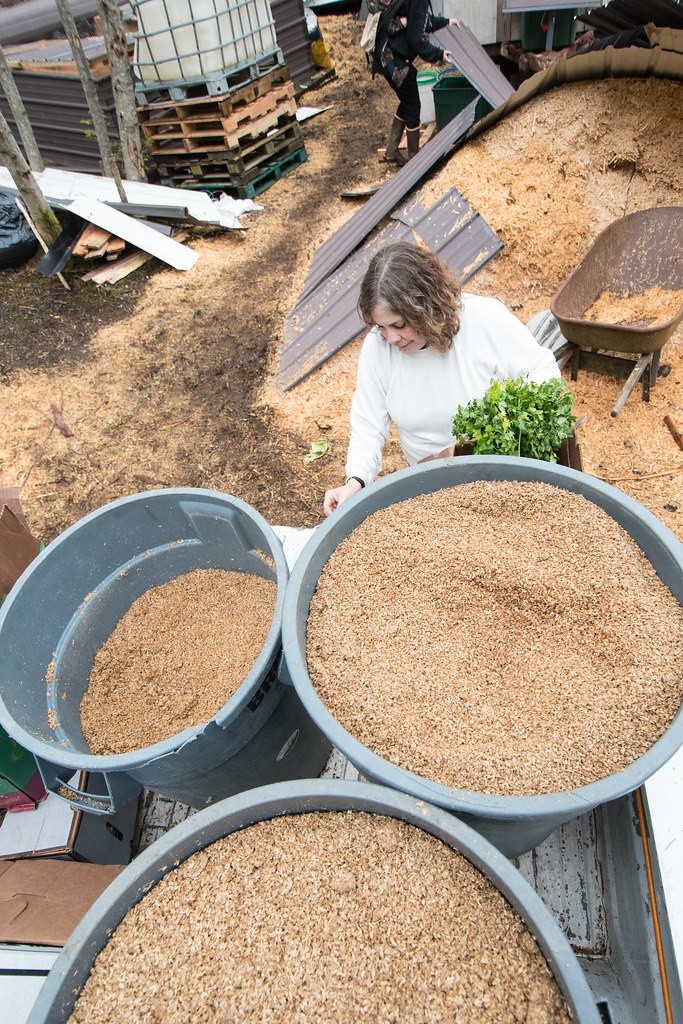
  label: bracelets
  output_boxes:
[347,476,365,489]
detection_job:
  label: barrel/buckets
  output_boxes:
[0,487,334,815]
[282,454,683,860]
[24,777,603,1024]
[416,71,439,123]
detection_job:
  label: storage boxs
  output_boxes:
[415,427,583,472]
[520,6,575,51]
[430,75,487,132]
[0,487,41,600]
[0,723,138,946]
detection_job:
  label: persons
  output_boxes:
[323,242,563,517]
[372,0,460,168]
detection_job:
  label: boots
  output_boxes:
[406,124,421,158]
[383,113,408,166]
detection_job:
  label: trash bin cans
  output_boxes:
[432,71,492,132]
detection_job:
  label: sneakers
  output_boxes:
[392,65,409,88]
